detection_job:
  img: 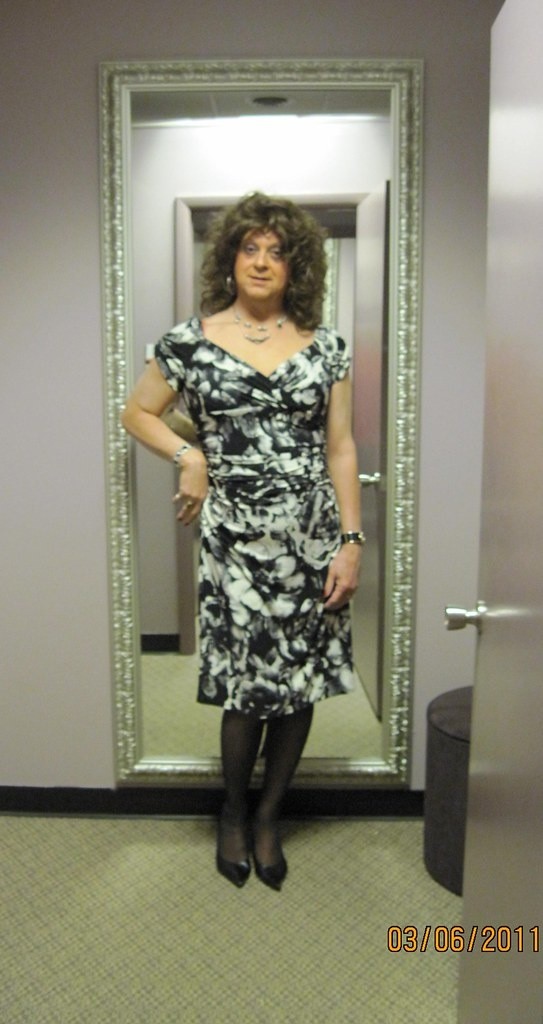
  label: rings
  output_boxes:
[186,501,193,509]
[176,495,180,498]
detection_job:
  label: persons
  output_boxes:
[121,188,367,892]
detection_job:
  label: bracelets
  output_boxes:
[173,444,193,464]
[341,531,367,544]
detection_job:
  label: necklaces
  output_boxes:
[232,311,289,344]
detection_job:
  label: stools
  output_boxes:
[424,685,474,898]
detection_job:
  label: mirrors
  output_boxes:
[97,56,425,790]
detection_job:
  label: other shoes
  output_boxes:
[252,820,288,891]
[216,811,251,888]
